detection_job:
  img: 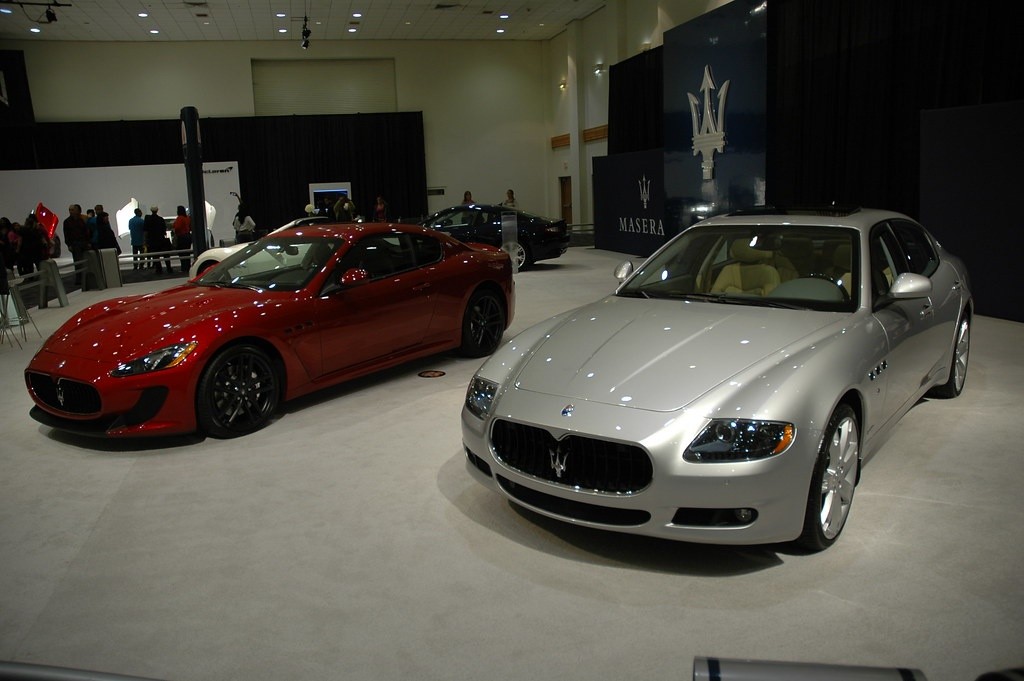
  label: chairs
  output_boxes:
[706,237,779,300]
[814,236,886,300]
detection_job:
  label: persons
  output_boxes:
[233,203,255,242]
[372,195,390,223]
[63,204,122,286]
[129,206,191,275]
[461,191,476,223]
[333,192,355,223]
[503,189,519,210]
[0,213,61,280]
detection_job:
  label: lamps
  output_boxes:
[301,15,312,49]
[46,5,58,24]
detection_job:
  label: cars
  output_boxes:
[415,202,571,271]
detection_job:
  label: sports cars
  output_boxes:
[23,218,514,441]
[188,226,401,288]
[460,204,976,555]
